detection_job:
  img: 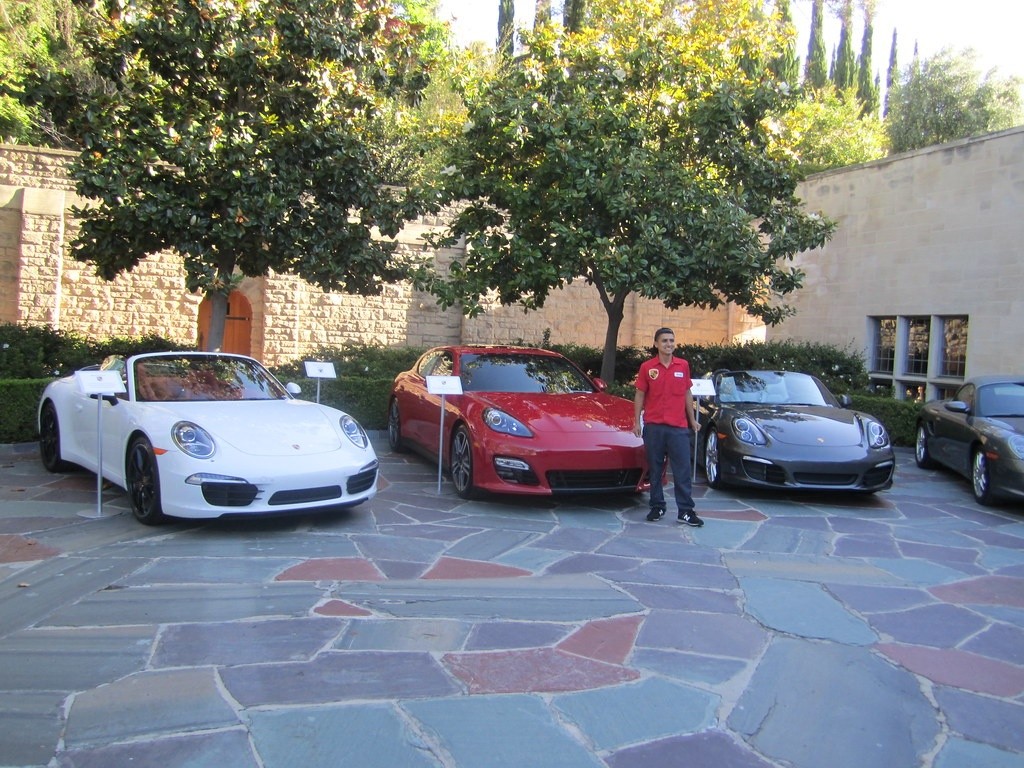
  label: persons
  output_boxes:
[632,327,704,527]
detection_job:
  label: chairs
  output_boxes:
[709,369,740,401]
[761,375,791,403]
[124,361,250,401]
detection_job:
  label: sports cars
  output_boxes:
[387,343,668,500]
[36,350,379,527]
[915,374,1024,507]
[687,369,896,494]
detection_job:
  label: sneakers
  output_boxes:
[646,508,665,521]
[677,509,704,526]
